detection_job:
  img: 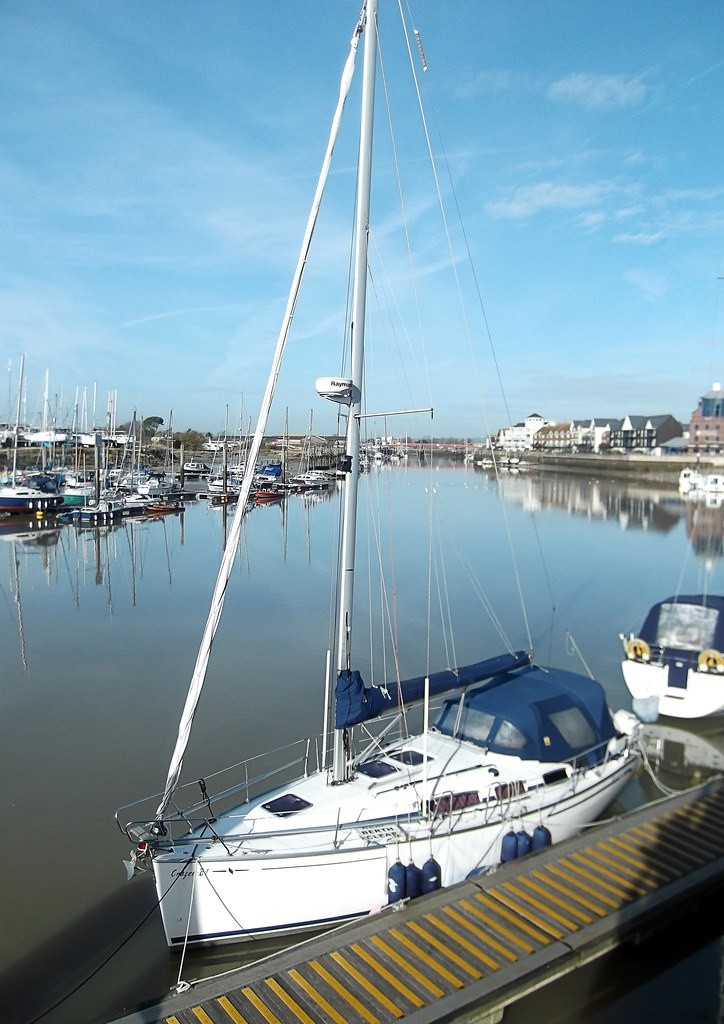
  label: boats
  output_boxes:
[482,457,492,465]
[679,467,722,496]
[73,518,121,586]
[71,433,124,523]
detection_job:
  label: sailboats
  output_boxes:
[112,0,649,956]
[618,274,723,724]
[0,511,65,670]
[0,353,66,513]
[0,368,408,515]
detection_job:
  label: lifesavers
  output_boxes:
[626,638,652,662]
[698,648,724,673]
